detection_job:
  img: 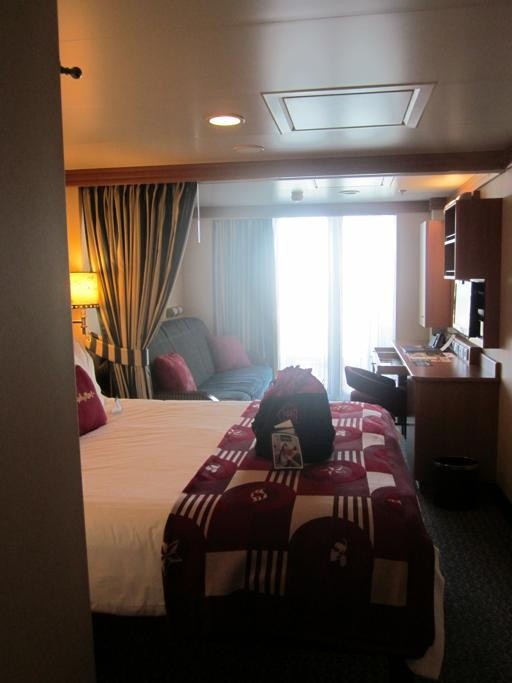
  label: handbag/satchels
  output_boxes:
[250,366,336,464]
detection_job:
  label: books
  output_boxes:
[400,345,436,353]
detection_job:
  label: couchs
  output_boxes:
[148,317,274,401]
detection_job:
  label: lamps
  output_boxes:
[70,272,100,336]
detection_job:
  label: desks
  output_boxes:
[368,340,502,489]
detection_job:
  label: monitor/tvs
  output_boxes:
[452,280,477,338]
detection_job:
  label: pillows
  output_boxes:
[73,338,104,407]
[154,351,196,391]
[76,365,107,437]
[207,335,251,371]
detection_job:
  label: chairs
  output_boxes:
[345,366,407,440]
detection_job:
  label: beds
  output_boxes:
[80,398,445,683]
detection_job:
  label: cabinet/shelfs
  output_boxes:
[444,199,504,349]
[420,221,453,329]
